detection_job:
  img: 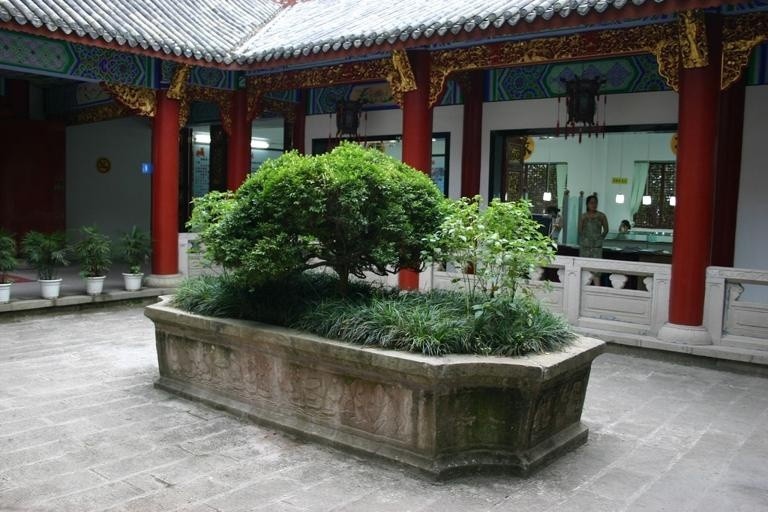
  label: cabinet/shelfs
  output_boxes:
[556,231,673,290]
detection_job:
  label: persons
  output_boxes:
[617,219,632,234]
[577,195,608,286]
[545,206,566,240]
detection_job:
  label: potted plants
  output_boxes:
[64,224,114,296]
[20,231,71,299]
[0,233,19,303]
[111,225,157,291]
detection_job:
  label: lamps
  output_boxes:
[542,131,677,206]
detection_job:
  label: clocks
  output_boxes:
[96,158,111,173]
